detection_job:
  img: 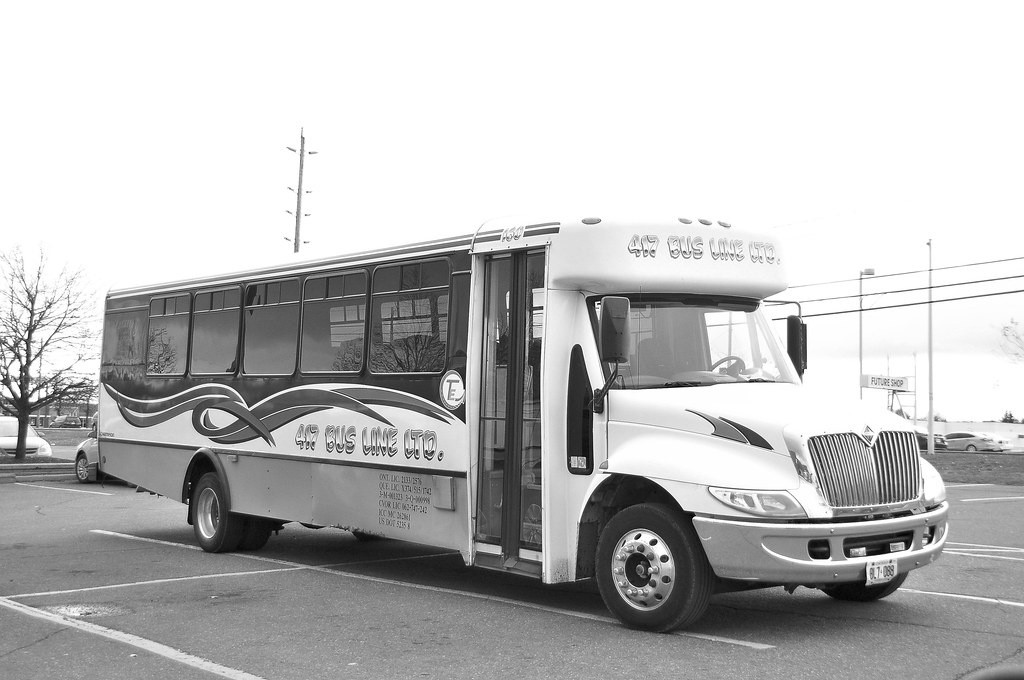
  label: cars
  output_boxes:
[92,411,99,430]
[943,431,1012,453]
[74,428,139,487]
[915,429,947,451]
[0,415,52,459]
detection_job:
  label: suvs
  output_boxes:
[48,415,82,429]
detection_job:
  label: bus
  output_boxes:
[96,213,952,632]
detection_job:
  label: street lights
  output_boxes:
[859,269,876,399]
[284,146,317,253]
